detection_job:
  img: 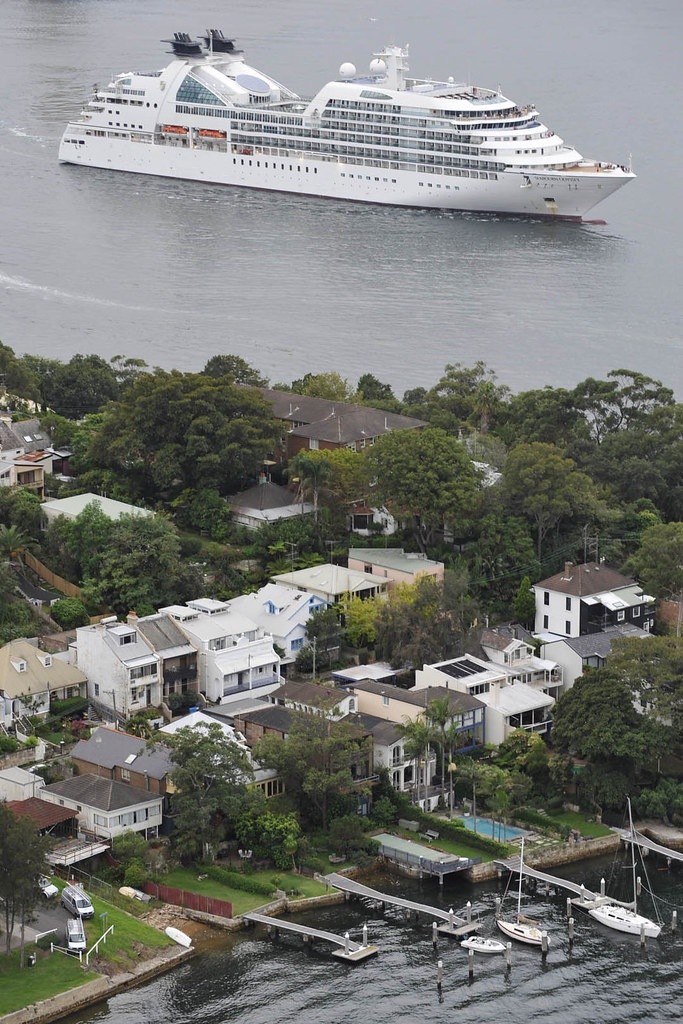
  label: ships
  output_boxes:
[56,25,638,222]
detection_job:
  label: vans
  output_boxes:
[61,888,95,920]
[64,919,88,952]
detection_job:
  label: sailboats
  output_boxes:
[495,836,551,944]
[587,797,665,938]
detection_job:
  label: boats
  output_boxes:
[460,936,507,953]
[195,131,226,146]
[165,927,192,950]
[162,125,189,140]
[134,889,151,903]
[119,886,137,900]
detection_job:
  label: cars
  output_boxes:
[37,876,60,899]
